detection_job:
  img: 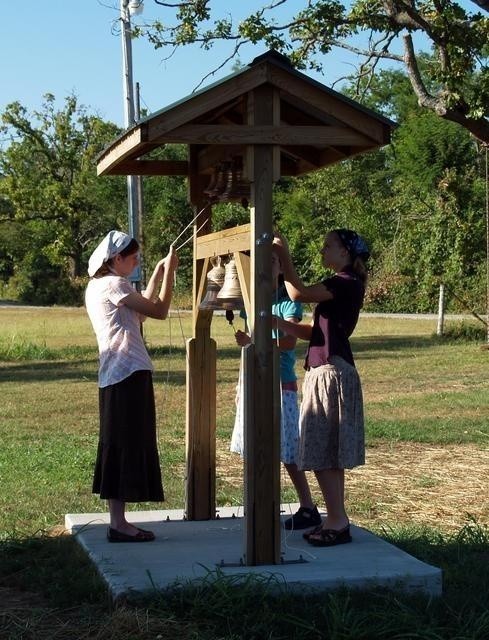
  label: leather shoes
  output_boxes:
[107,525,154,542]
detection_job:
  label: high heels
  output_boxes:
[303,525,351,546]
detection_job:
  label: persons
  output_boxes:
[85,231,179,543]
[274,229,368,545]
[229,246,323,530]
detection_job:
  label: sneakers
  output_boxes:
[285,506,322,529]
